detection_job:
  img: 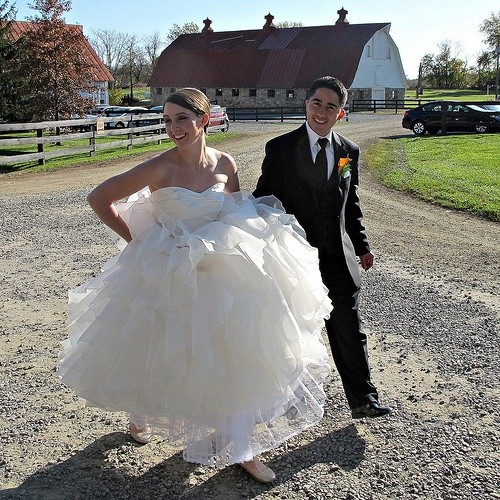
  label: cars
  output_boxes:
[81,104,229,133]
[402,101,500,135]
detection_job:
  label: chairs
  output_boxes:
[445,105,459,111]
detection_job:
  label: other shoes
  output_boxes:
[130,423,152,444]
[238,458,276,483]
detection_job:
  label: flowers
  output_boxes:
[338,158,352,179]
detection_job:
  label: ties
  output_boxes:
[315,138,328,194]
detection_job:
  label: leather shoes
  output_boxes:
[352,403,393,419]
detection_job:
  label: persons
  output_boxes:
[251,75,392,419]
[86,88,276,486]
